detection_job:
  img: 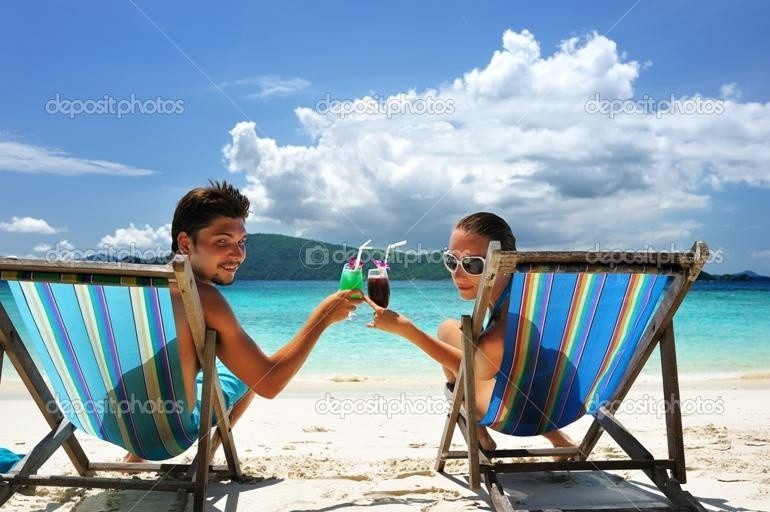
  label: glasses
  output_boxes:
[440,248,489,279]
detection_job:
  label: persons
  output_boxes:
[361,210,577,463]
[119,180,365,477]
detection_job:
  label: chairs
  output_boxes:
[435,239,709,511]
[0,255,242,512]
